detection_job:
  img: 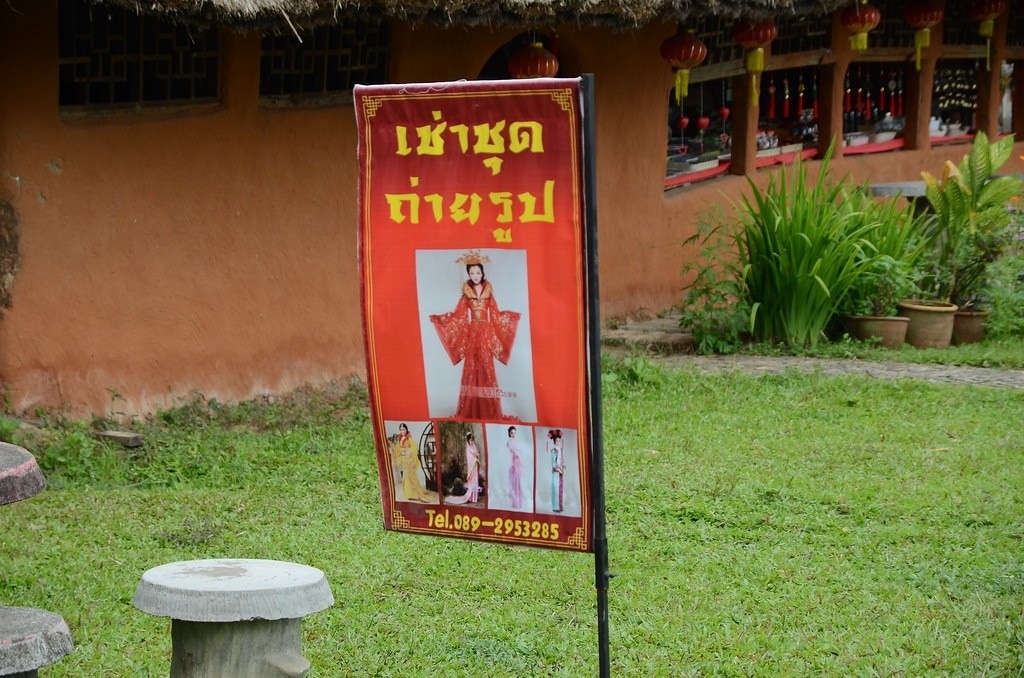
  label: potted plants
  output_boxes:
[885,128,1021,349]
[838,195,940,349]
[949,207,1011,346]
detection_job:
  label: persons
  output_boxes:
[393,423,420,500]
[430,262,520,420]
[551,436,565,513]
[466,434,480,502]
[505,426,523,508]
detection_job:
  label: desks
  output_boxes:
[0,430,49,517]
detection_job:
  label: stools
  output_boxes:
[0,602,75,677]
[132,556,334,678]
